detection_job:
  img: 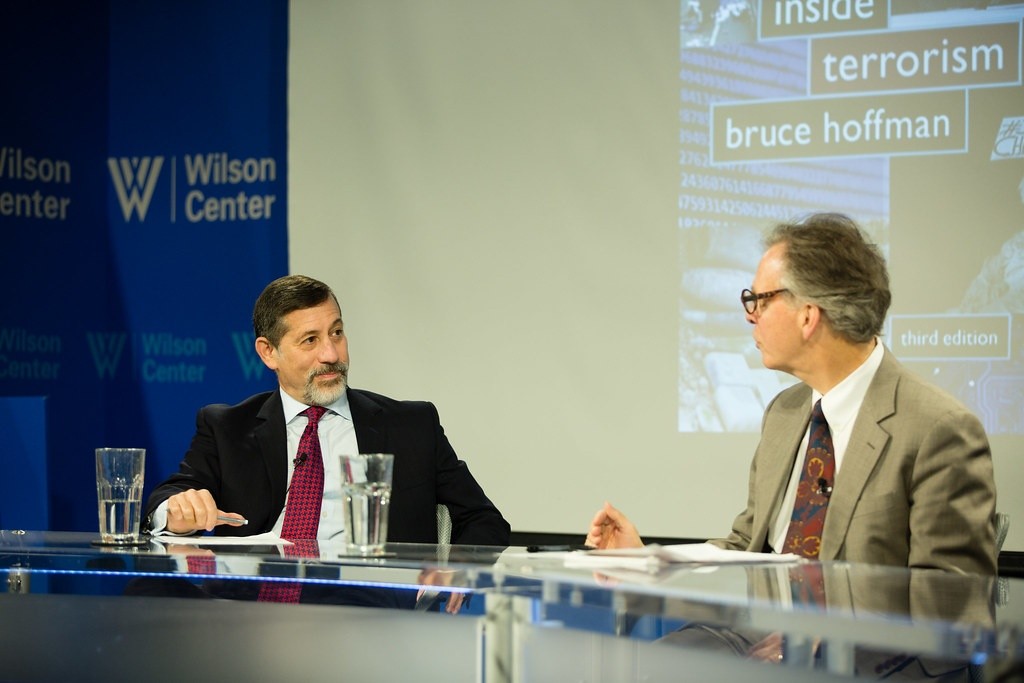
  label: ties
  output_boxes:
[780,397,836,560]
[280,406,329,539]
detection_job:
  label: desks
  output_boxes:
[0,530,1024,682]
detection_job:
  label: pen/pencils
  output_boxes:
[527,544,598,552]
[166,508,248,525]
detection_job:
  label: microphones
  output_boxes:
[817,478,828,494]
[294,452,307,469]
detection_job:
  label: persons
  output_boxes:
[141,274,513,616]
[584,212,999,682]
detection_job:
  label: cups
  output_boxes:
[95,448,145,545]
[340,453,394,553]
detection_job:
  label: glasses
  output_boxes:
[740,288,788,315]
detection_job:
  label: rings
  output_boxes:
[775,653,784,661]
